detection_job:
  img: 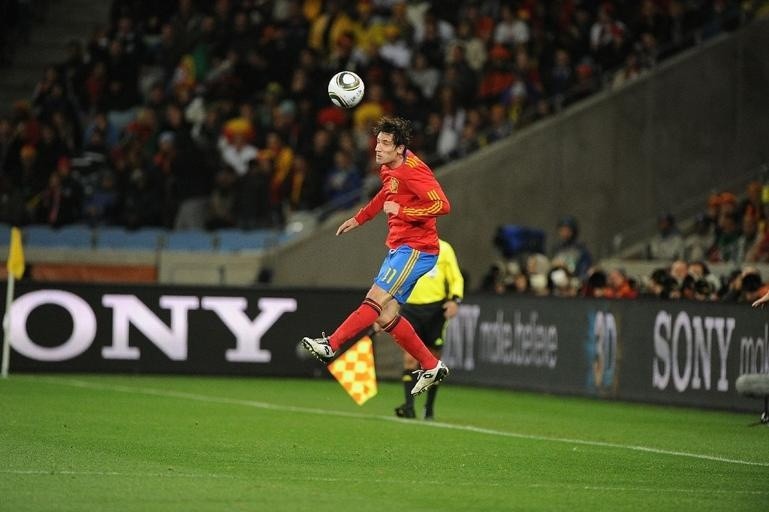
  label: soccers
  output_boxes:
[328,71,365,108]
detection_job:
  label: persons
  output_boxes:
[374,238,465,419]
[302,115,450,396]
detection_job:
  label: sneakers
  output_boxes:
[301,336,336,367]
[395,403,416,418]
[410,359,450,397]
